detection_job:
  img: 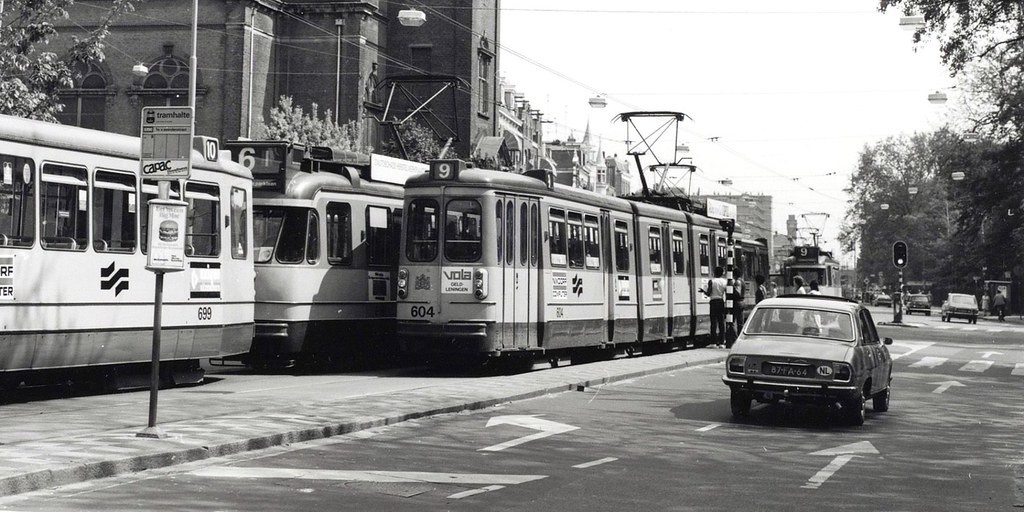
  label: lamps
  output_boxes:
[928,91,948,104]
[589,95,607,108]
[899,16,926,31]
[908,187,918,194]
[952,172,965,180]
[677,144,690,153]
[881,204,889,210]
[397,7,427,26]
[132,63,149,77]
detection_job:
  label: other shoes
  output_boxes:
[706,344,716,348]
[719,344,726,349]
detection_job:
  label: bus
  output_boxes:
[222,73,774,376]
[781,212,843,297]
[0,115,255,393]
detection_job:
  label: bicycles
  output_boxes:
[992,302,1005,322]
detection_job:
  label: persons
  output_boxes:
[865,288,1007,321]
[698,265,823,349]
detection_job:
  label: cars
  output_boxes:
[905,293,931,316]
[941,292,979,324]
[849,287,893,308]
[721,294,893,426]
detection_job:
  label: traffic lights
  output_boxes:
[892,239,909,270]
